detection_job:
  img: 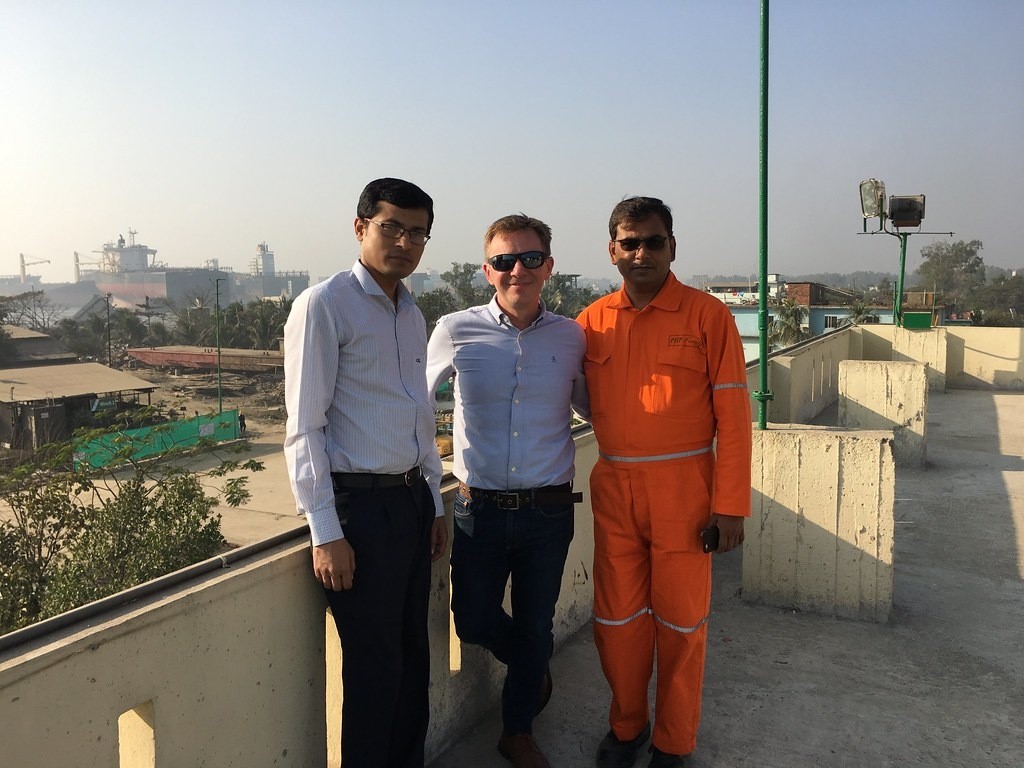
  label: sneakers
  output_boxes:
[596,724,650,767]
[648,743,693,767]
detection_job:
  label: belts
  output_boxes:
[331,465,424,491]
[458,481,583,511]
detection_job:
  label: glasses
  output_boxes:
[487,251,549,272]
[363,216,431,246]
[613,235,672,251]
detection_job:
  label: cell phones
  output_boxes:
[703,526,719,553]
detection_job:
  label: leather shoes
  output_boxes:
[498,730,550,768]
[531,634,554,717]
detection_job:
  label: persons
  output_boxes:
[238,410,247,433]
[423,212,589,768]
[575,197,752,768]
[283,176,451,768]
